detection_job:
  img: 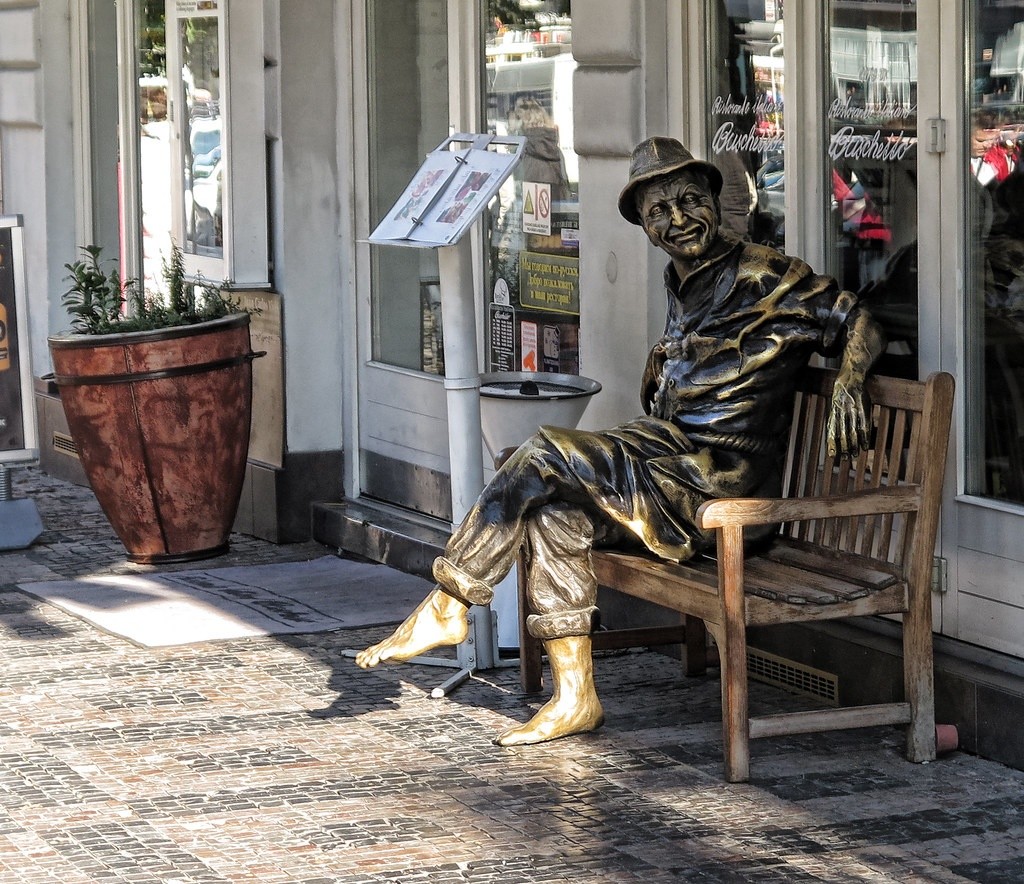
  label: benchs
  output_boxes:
[493,363,955,781]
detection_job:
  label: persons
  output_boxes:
[970,107,1016,184]
[356,138,884,746]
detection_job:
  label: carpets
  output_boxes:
[8,553,437,650]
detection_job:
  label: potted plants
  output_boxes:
[41,229,266,565]
